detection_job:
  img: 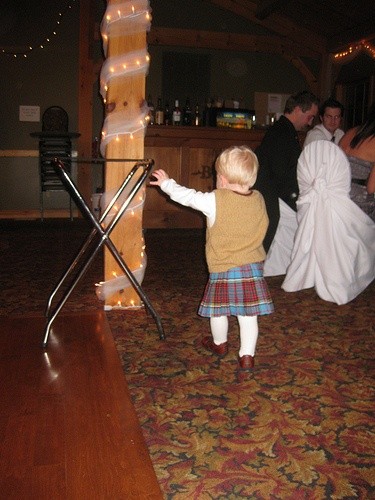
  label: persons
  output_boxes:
[304,98,345,144]
[339,102,375,222]
[150,146,274,370]
[253,91,321,251]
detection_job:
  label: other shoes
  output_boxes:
[239,355,255,373]
[202,335,228,358]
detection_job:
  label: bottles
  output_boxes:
[172,97,183,125]
[147,95,155,126]
[164,103,171,125]
[182,97,192,126]
[191,104,203,127]
[155,96,164,126]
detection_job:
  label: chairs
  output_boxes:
[38,105,74,224]
